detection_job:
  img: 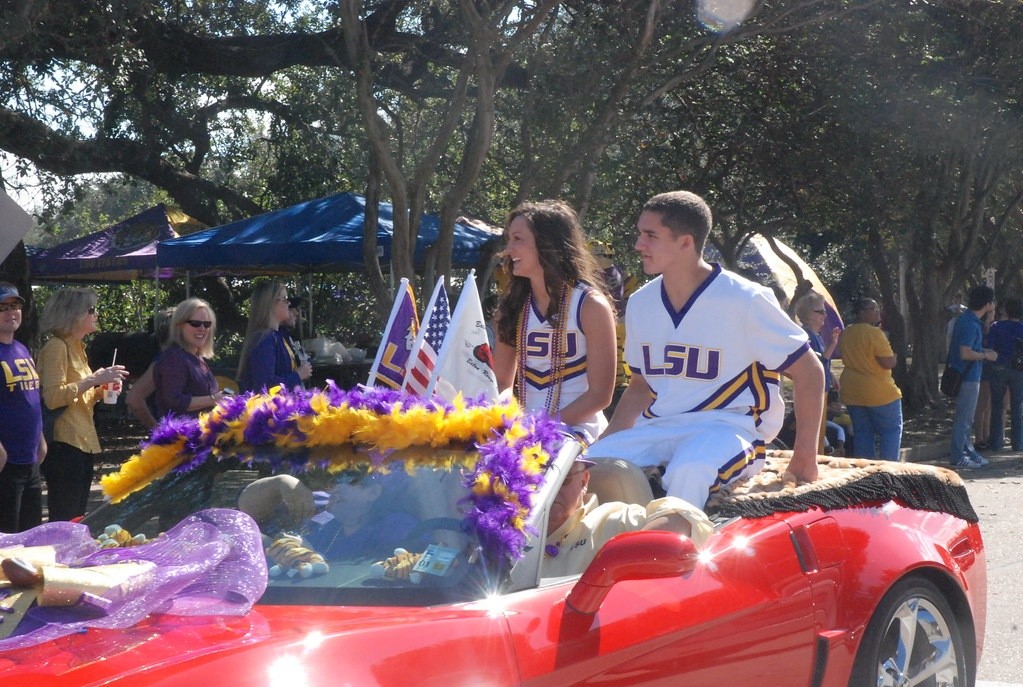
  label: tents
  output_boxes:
[155,191,501,335]
[25,204,299,299]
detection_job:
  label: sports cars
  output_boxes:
[1,437,987,687]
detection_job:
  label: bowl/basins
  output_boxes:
[347,348,367,361]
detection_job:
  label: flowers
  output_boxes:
[97,387,580,575]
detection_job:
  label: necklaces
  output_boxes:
[544,533,568,559]
[516,283,573,415]
[323,525,343,557]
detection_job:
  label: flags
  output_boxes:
[376,274,499,401]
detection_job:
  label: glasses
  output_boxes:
[186,320,212,328]
[84,305,97,314]
[0,302,21,312]
[276,297,290,304]
[813,309,826,314]
[562,470,583,486]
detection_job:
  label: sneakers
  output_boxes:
[966,456,988,465]
[951,456,980,469]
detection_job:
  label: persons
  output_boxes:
[947,286,998,468]
[794,293,841,455]
[493,200,617,452]
[777,389,852,457]
[985,298,1023,451]
[236,280,313,395]
[470,453,715,579]
[584,190,825,510]
[0,283,48,534]
[838,298,902,462]
[482,295,499,354]
[303,472,431,564]
[36,286,129,523]
[126,299,225,533]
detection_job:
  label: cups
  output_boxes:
[103,366,122,404]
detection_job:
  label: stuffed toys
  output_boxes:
[370,548,424,584]
[96,525,165,551]
[266,537,329,578]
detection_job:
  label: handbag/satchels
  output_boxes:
[941,367,962,397]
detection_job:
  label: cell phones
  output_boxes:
[222,387,234,395]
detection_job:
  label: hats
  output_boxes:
[0,282,25,304]
[573,445,597,470]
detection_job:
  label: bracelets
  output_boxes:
[826,447,834,455]
[211,394,214,400]
